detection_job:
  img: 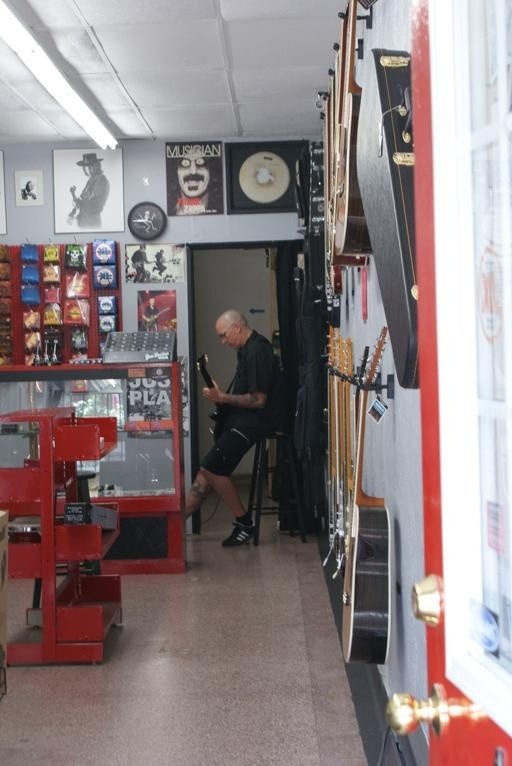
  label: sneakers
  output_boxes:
[221,521,256,548]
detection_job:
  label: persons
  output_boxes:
[131,244,151,283]
[66,152,111,227]
[142,298,160,332]
[169,143,219,215]
[151,249,167,277]
[181,307,289,548]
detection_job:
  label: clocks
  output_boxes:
[127,202,167,241]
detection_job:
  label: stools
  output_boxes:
[245,431,308,547]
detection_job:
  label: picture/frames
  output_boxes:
[225,141,310,215]
[52,148,125,235]
[14,169,44,207]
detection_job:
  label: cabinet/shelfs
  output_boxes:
[0,405,122,667]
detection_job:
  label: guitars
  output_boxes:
[43,339,49,363]
[195,352,230,442]
[33,340,40,363]
[323,326,392,665]
[50,340,58,361]
[316,0,375,308]
[142,306,171,328]
